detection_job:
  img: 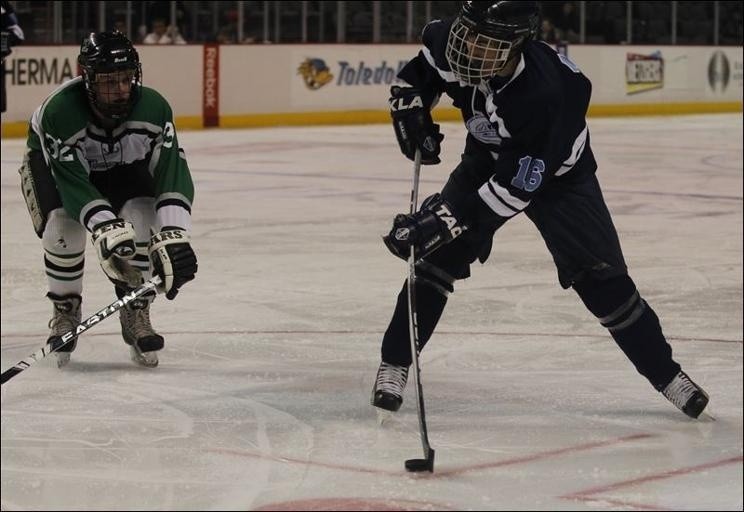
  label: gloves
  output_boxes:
[379,191,470,266]
[87,218,156,292]
[390,89,446,166]
[148,228,204,306]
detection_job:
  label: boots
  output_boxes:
[45,290,83,354]
[115,300,166,352]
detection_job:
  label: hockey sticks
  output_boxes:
[405,146,435,474]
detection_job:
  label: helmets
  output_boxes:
[453,1,541,62]
[77,31,139,90]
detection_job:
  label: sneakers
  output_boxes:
[662,374,711,421]
[371,363,411,414]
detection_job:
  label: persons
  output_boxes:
[367,0,710,422]
[14,28,200,356]
